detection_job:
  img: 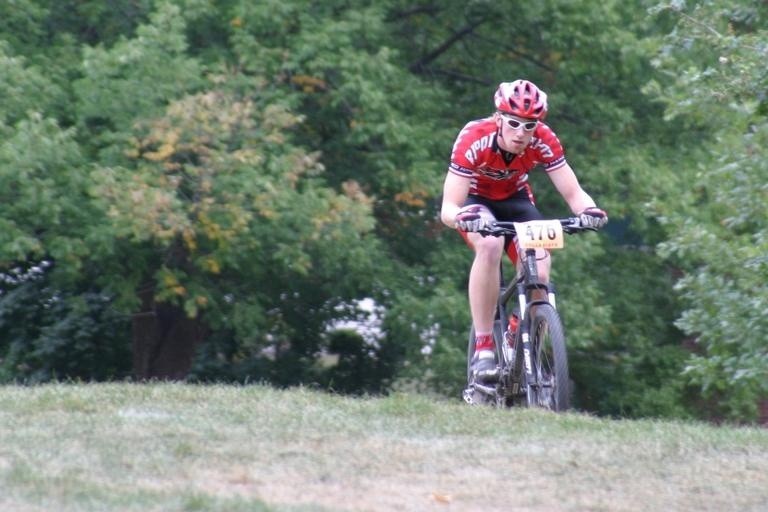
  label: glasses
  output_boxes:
[500,115,538,132]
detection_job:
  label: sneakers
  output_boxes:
[467,350,499,381]
[501,332,522,368]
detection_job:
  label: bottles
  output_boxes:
[504,308,520,361]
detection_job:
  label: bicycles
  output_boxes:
[453,215,598,414]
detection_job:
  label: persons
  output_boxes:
[440,79,609,386]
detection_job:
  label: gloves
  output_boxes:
[578,206,609,229]
[455,209,485,233]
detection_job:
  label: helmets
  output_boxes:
[494,78,549,120]
